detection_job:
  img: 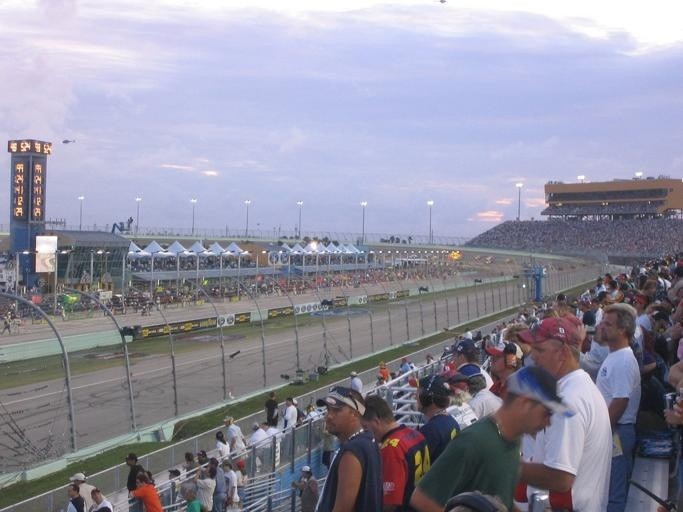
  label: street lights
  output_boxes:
[427,200,434,244]
[97,251,103,293]
[576,174,585,183]
[255,250,262,297]
[295,199,302,238]
[176,250,185,297]
[190,197,197,236]
[76,196,83,232]
[271,251,280,296]
[287,251,296,294]
[236,250,246,298]
[299,250,447,290]
[121,250,136,315]
[150,250,167,303]
[15,251,30,315]
[242,198,251,238]
[359,200,367,243]
[634,172,642,179]
[134,197,143,232]
[515,182,522,222]
[53,250,68,314]
[195,250,206,303]
[219,250,228,296]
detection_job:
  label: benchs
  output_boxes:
[620,448,673,512]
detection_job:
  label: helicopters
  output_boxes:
[61,138,76,144]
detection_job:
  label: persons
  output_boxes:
[1,191,683,511]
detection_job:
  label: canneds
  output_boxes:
[663,392,681,432]
[528,491,549,512]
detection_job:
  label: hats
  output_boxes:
[503,365,577,418]
[316,385,366,419]
[445,338,481,356]
[514,317,583,347]
[65,414,261,484]
